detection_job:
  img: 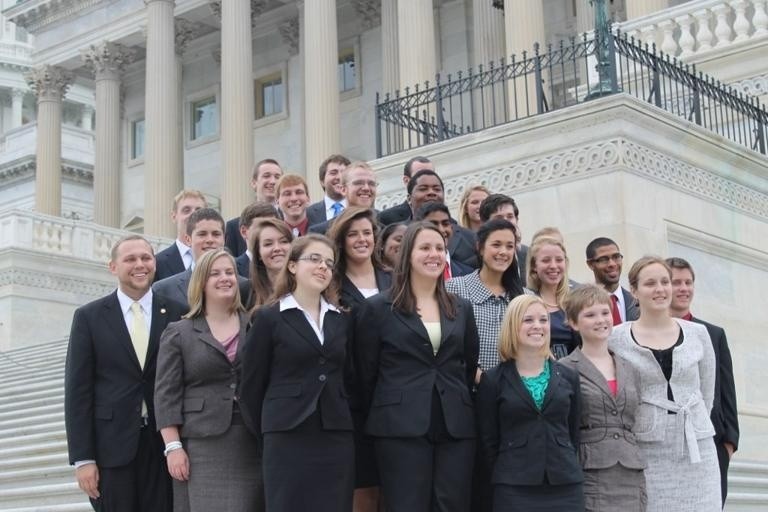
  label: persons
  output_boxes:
[475,293,586,511]
[665,256,740,503]
[155,248,262,512]
[234,201,283,277]
[465,193,530,280]
[153,188,207,281]
[374,221,409,271]
[245,219,295,329]
[378,155,435,226]
[607,255,723,511]
[585,236,641,328]
[306,160,382,234]
[225,158,282,258]
[275,174,316,238]
[326,206,392,310]
[153,207,249,309]
[413,201,473,280]
[305,154,351,226]
[459,185,491,230]
[375,170,477,264]
[525,235,582,360]
[555,285,648,511]
[64,235,190,512]
[444,216,536,390]
[239,232,353,512]
[350,219,481,511]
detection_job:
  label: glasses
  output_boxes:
[342,181,380,187]
[296,252,335,270]
[588,254,623,264]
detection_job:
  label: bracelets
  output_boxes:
[163,441,184,457]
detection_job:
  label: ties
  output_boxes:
[443,262,450,280]
[332,202,343,217]
[610,295,623,326]
[130,302,150,417]
[292,227,300,238]
